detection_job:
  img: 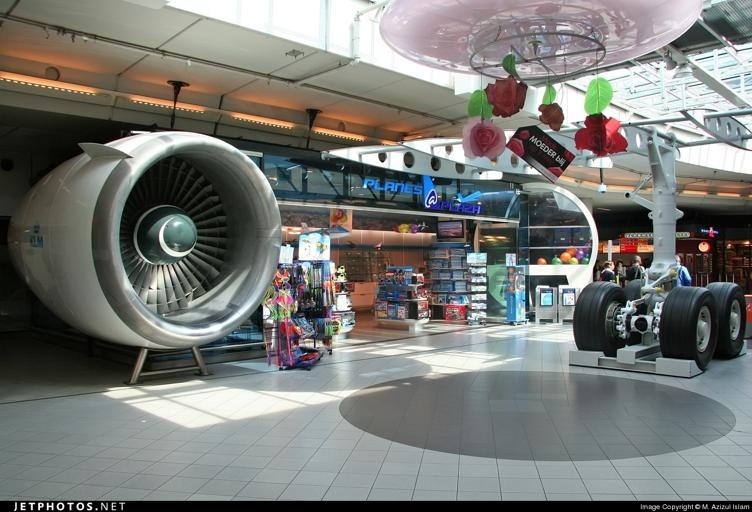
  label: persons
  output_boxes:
[615,259,627,279]
[600,261,615,283]
[629,256,644,280]
[593,260,603,282]
[499,266,518,321]
[674,254,692,288]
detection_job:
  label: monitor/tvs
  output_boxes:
[435,220,465,240]
[562,288,576,307]
[538,288,554,307]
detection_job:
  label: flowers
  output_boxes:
[463,37,628,161]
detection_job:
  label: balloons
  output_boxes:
[391,224,399,232]
[399,224,409,233]
[331,208,347,224]
[409,223,419,234]
[536,247,591,265]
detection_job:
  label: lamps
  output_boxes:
[379,0,703,86]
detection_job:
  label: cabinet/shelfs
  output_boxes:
[332,242,472,334]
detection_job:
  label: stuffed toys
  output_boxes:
[335,264,348,281]
